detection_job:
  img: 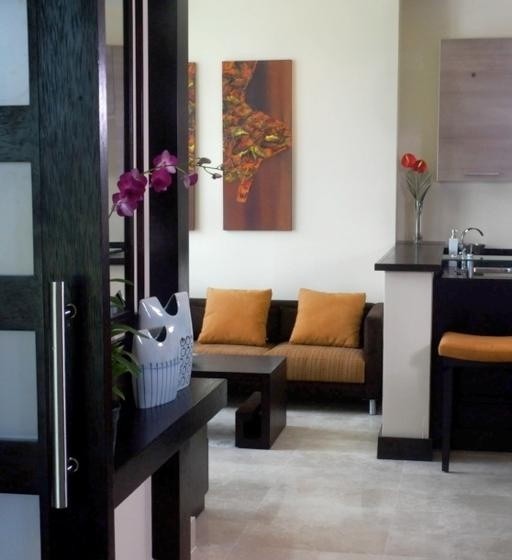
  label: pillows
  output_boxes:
[197,287,273,348]
[287,288,366,347]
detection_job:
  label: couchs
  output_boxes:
[187,298,383,415]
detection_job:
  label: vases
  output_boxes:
[414,201,422,244]
[131,323,179,409]
[139,291,193,390]
[110,333,126,356]
[112,404,121,461]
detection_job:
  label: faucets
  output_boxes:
[458,228,484,255]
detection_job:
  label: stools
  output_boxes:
[437,332,512,472]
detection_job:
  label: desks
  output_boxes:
[111,378,228,560]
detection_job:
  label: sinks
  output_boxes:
[474,267,511,274]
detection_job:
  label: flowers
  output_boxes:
[109,151,250,220]
[401,152,433,201]
